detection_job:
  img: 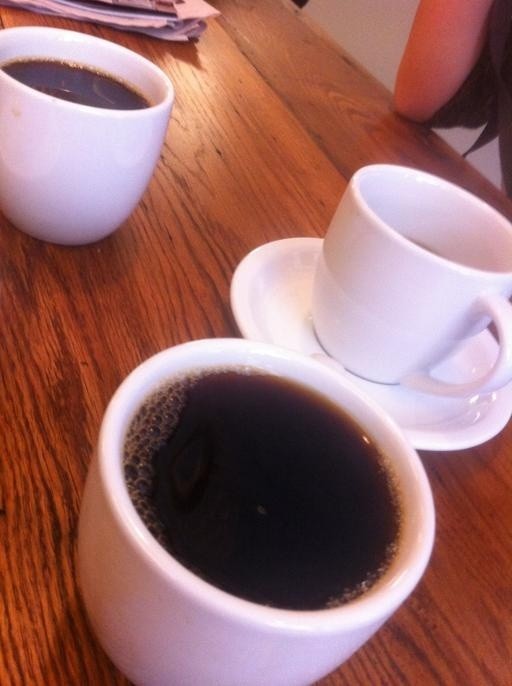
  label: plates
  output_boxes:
[230,233,512,452]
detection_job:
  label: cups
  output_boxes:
[76,338,438,686]
[308,162,512,398]
[0,26,174,248]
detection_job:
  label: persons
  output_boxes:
[391,0,512,200]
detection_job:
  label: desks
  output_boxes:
[3,0,512,686]
